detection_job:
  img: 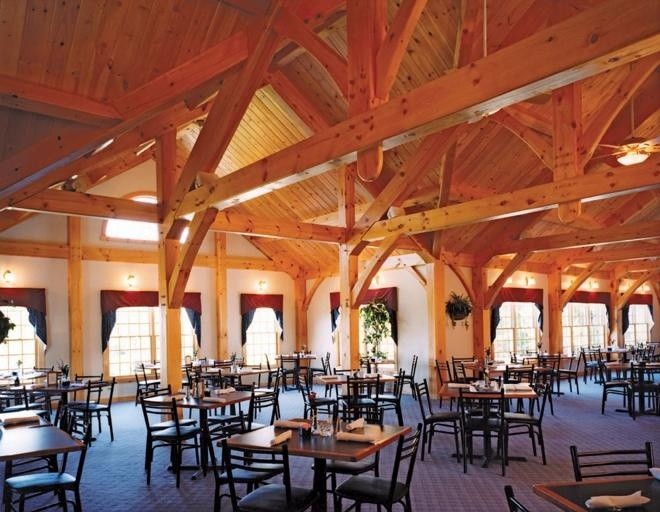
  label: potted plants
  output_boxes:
[447,291,473,329]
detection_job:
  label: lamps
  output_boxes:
[617,100,650,167]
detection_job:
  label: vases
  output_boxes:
[61,376,70,388]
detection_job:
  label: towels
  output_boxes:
[584,489,651,511]
[70,383,87,386]
[447,381,472,389]
[346,416,366,430]
[216,386,237,395]
[335,431,376,445]
[203,395,227,403]
[321,375,338,381]
[4,415,39,426]
[272,418,310,432]
[178,388,193,395]
[271,429,293,446]
[505,384,533,392]
[647,467,660,481]
[164,394,183,404]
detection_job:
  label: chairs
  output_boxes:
[139,384,199,470]
[321,356,344,397]
[280,355,300,393]
[4,416,92,512]
[296,374,337,420]
[597,358,631,415]
[221,388,280,434]
[504,484,530,511]
[498,383,549,466]
[12,400,68,495]
[581,347,597,384]
[393,354,418,401]
[458,387,509,477]
[139,396,200,488]
[452,363,473,409]
[554,351,581,398]
[68,372,103,433]
[505,364,534,414]
[627,361,660,421]
[70,377,115,447]
[221,438,313,512]
[342,374,379,423]
[132,363,160,406]
[569,440,654,482]
[336,422,423,512]
[371,368,405,426]
[264,354,282,388]
[435,360,455,412]
[415,378,463,463]
[200,411,280,512]
[510,353,519,363]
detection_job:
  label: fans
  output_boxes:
[592,98,660,171]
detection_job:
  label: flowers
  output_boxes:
[57,358,71,376]
